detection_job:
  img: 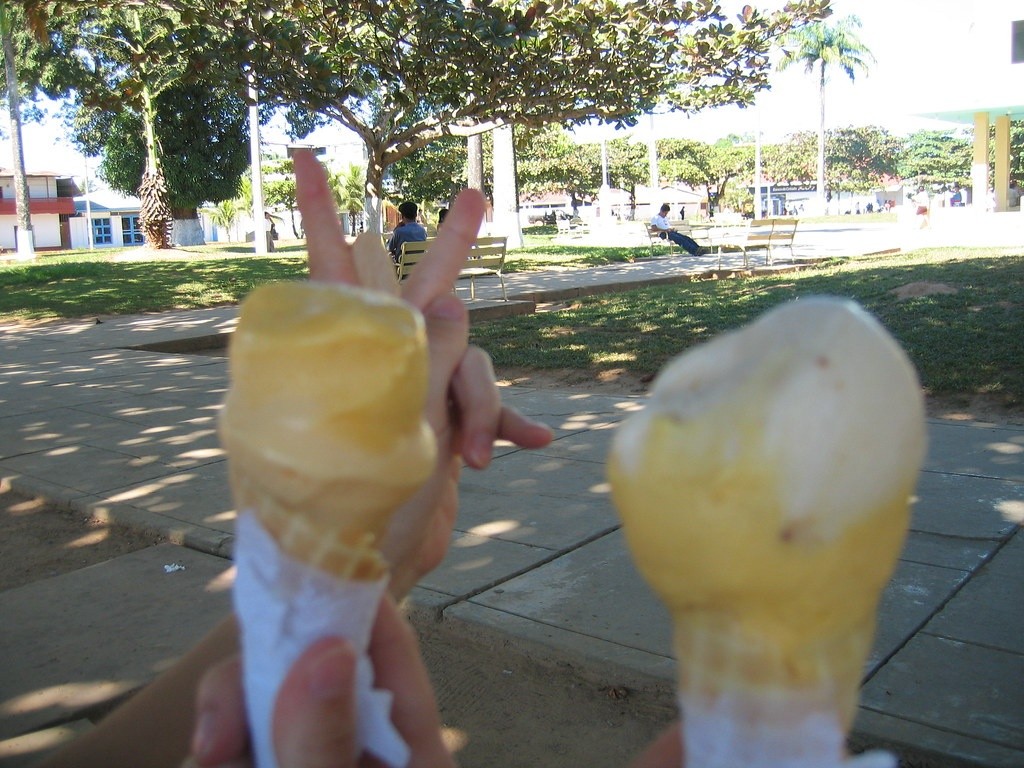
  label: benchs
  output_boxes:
[644,221,715,259]
[740,218,798,266]
[556,220,582,239]
[399,236,509,301]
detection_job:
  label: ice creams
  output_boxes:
[608,293,927,768]
[216,276,439,768]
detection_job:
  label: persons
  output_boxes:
[653,204,707,255]
[913,185,930,232]
[389,201,427,282]
[679,206,685,220]
[0,149,552,768]
[846,198,894,215]
[433,205,482,261]
[784,203,806,216]
